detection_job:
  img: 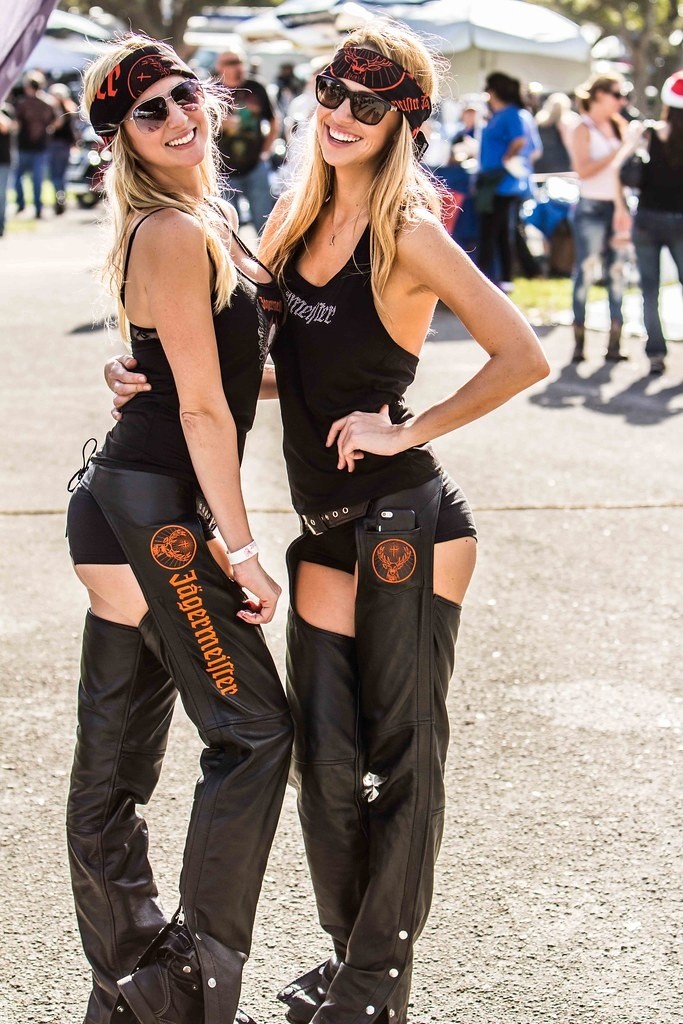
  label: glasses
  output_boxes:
[122,78,207,135]
[315,74,402,126]
[607,89,630,100]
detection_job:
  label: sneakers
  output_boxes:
[650,361,665,376]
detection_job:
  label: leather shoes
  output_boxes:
[117,927,205,1023]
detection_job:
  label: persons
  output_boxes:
[422,71,653,294]
[570,75,640,363]
[619,72,683,376]
[105,26,551,1024]
[0,70,82,239]
[65,35,296,1024]
[203,52,329,247]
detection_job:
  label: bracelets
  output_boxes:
[225,538,259,566]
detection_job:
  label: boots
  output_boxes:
[605,330,628,361]
[573,324,585,362]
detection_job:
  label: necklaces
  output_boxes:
[329,183,370,246]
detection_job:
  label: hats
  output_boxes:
[660,70,683,110]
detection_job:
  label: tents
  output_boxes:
[182,0,593,103]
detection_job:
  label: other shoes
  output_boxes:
[37,209,40,217]
[56,199,64,215]
[17,206,23,213]
[497,280,515,293]
